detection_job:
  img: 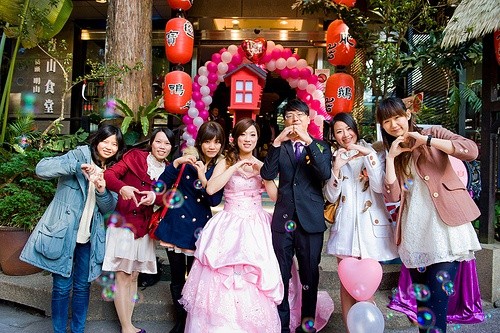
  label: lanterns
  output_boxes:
[165,0,195,115]
[326,0,356,115]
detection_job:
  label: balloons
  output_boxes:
[181,37,324,147]
[334,257,382,302]
[345,302,386,333]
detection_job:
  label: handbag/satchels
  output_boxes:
[324,195,340,223]
[148,206,166,240]
[138,257,162,291]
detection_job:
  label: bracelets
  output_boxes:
[427,135,432,146]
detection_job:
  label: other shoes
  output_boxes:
[136,328,146,333]
[175,319,186,333]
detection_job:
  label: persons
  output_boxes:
[20,99,481,333]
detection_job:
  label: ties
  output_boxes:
[294,142,303,161]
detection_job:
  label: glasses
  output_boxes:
[285,113,308,120]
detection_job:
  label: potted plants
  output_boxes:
[0,149,60,276]
